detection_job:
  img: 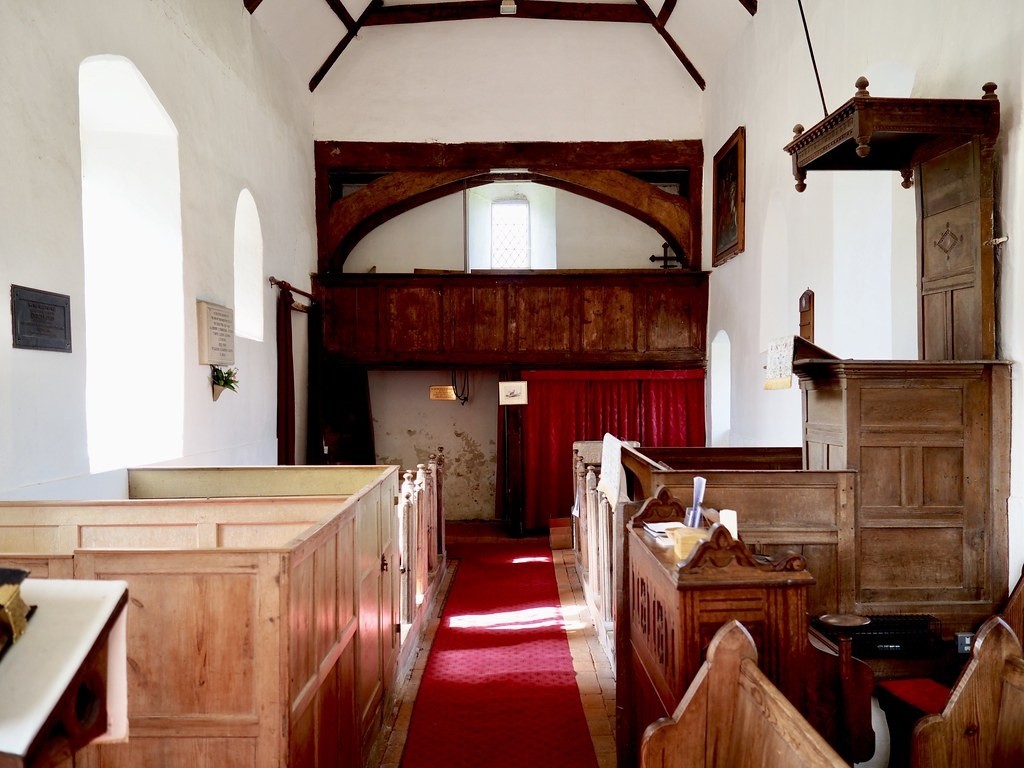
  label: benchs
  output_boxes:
[878,674,951,768]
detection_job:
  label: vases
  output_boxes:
[212,385,226,400]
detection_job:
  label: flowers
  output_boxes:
[208,363,239,393]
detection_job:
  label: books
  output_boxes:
[642,521,687,546]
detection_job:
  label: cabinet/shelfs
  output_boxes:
[625,484,816,768]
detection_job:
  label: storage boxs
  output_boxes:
[674,528,707,560]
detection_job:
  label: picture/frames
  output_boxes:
[712,126,745,268]
